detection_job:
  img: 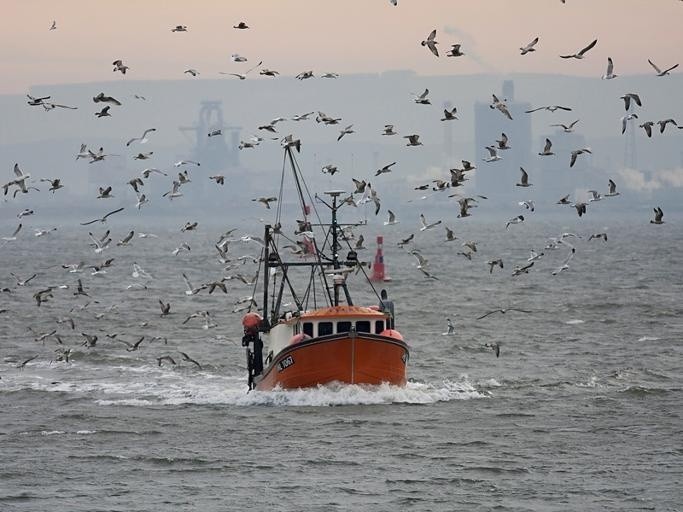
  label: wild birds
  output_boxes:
[108,55,339,80]
[171,25,189,33]
[3,91,366,371]
[49,20,58,31]
[442,306,533,360]
[233,22,250,29]
[560,38,599,60]
[354,88,620,284]
[622,91,683,138]
[421,29,440,58]
[602,58,678,81]
[519,37,539,56]
[646,204,667,224]
[447,44,464,58]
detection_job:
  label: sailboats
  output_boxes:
[240,132,410,392]
